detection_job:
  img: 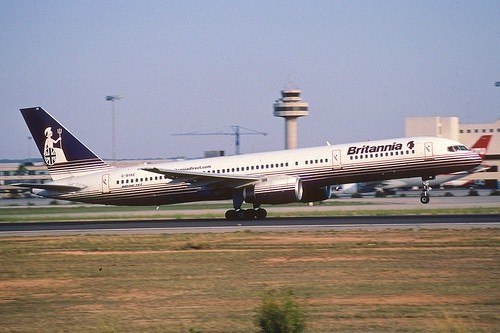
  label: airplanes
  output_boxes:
[10,106,481,220]
[329,134,492,196]
[397,177,499,196]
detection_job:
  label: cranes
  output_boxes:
[171,122,267,154]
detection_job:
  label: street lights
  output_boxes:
[105,93,124,159]
